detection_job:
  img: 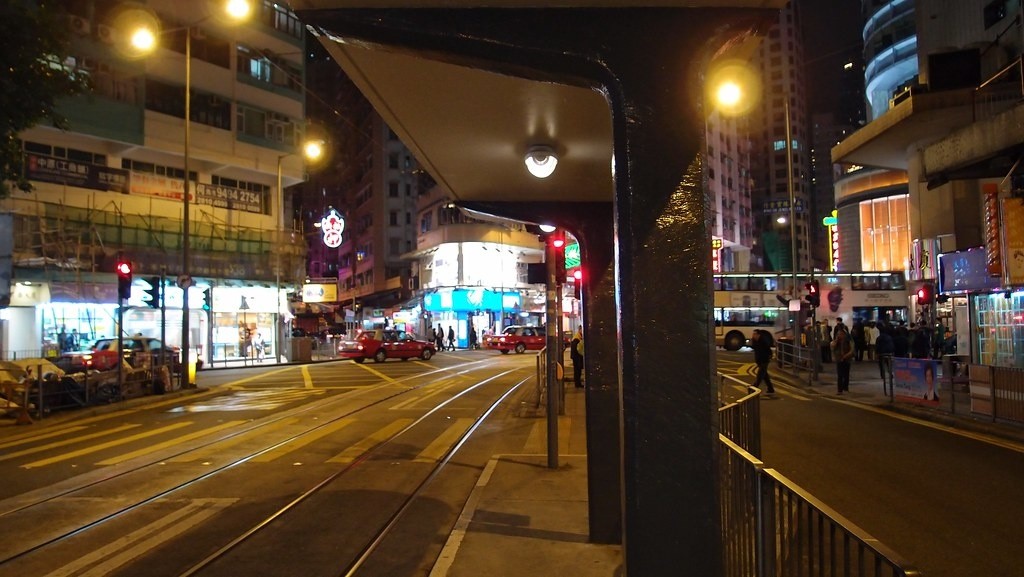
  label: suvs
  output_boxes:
[89,336,179,374]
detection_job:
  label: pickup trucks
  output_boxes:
[483,325,572,354]
[337,330,436,364]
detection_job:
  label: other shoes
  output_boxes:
[837,386,848,395]
[575,384,584,388]
[765,391,775,396]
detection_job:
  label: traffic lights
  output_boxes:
[554,229,567,283]
[116,259,131,298]
[574,267,582,300]
[918,280,933,305]
[806,279,820,307]
[144,274,160,308]
[201,289,212,310]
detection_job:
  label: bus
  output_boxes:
[712,272,907,351]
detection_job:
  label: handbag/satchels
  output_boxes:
[452,338,455,342]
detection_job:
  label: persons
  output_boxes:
[470,327,480,350]
[922,363,939,400]
[58,327,79,351]
[751,329,774,394]
[426,323,455,352]
[830,329,850,395]
[805,317,945,380]
[254,333,264,364]
[482,326,494,336]
[571,326,585,389]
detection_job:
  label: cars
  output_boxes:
[0,345,122,410]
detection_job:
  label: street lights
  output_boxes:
[113,1,257,390]
[713,78,799,376]
[276,130,327,368]
